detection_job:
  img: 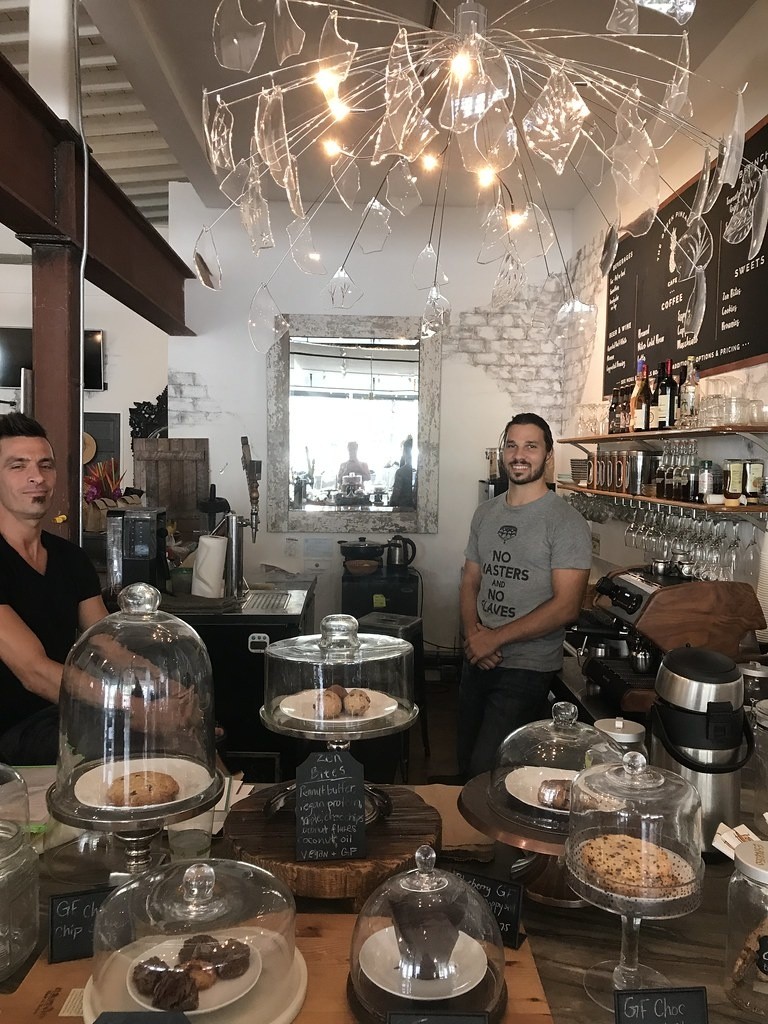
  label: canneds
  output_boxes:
[586,451,660,495]
[723,458,764,506]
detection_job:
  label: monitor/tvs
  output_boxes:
[0,327,103,392]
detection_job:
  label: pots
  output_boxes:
[337,538,401,559]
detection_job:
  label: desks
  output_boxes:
[0,657,768,1024]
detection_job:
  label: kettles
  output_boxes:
[387,536,416,567]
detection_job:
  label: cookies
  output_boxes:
[733,918,768,985]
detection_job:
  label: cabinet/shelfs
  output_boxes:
[103,573,316,783]
[342,566,418,617]
[557,424,768,533]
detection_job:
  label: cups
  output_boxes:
[754,522,768,644]
[698,395,764,427]
[587,451,661,495]
[171,570,192,595]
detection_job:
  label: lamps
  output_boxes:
[185,0,768,353]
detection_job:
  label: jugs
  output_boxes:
[647,645,756,858]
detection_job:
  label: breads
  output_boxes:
[579,835,676,897]
[537,779,596,811]
[316,684,372,719]
[109,772,179,804]
[132,935,251,1009]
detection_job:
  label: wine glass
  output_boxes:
[574,404,610,436]
[623,508,760,577]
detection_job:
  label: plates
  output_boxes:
[557,457,588,485]
[567,842,694,902]
[75,758,214,811]
[279,687,398,727]
[359,926,487,1000]
[125,937,261,1014]
[505,769,625,814]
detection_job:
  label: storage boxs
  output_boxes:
[358,612,423,652]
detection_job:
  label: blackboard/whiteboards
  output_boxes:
[49,887,135,964]
[294,751,366,859]
[614,986,708,1024]
[434,865,525,949]
[603,112,768,398]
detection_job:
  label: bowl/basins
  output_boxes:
[346,560,378,575]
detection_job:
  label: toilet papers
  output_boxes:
[190,534,229,599]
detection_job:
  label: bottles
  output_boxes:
[608,357,700,432]
[655,440,763,508]
[725,841,768,1014]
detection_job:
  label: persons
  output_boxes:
[453,412,592,785]
[339,441,370,486]
[0,412,232,780]
[374,462,400,486]
